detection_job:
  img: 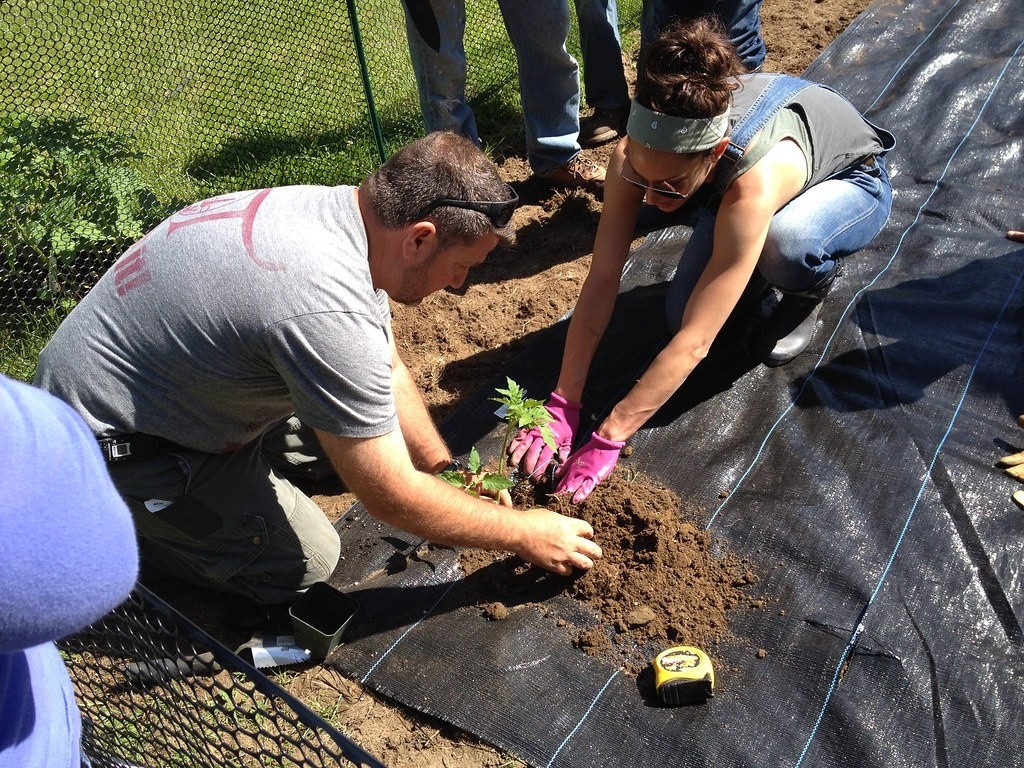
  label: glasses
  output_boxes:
[409,180,519,229]
[620,158,710,199]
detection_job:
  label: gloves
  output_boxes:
[551,429,626,504]
[507,392,583,481]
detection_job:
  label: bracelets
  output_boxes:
[438,459,462,477]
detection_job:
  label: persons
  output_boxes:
[32,130,604,604]
[507,14,896,505]
[0,373,140,768]
[398,0,612,192]
[575,0,762,145]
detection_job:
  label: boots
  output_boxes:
[756,257,842,365]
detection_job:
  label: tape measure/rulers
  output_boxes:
[652,645,717,706]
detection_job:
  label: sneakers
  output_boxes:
[581,99,632,142]
[537,152,607,195]
